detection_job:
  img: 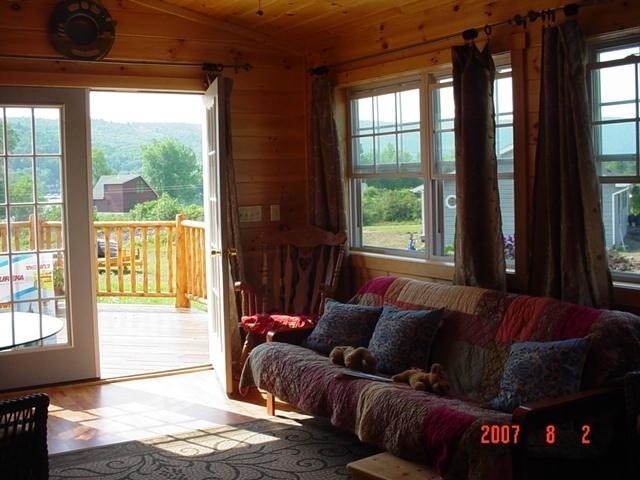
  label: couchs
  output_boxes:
[250,274,640,480]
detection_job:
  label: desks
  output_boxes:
[0,312,64,350]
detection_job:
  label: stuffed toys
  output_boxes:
[328,344,379,376]
[392,363,450,395]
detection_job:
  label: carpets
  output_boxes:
[48,412,386,480]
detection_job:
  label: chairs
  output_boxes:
[0,392,50,480]
[234,224,347,395]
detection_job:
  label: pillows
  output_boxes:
[364,301,447,375]
[302,297,383,356]
[481,333,594,414]
